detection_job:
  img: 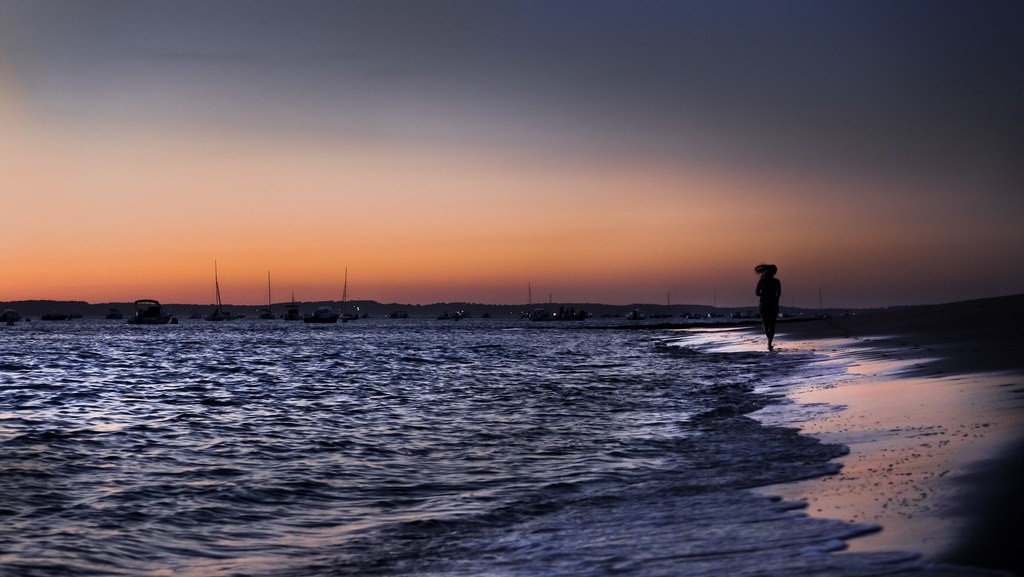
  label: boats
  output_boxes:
[258,264,358,324]
[389,311,410,319]
[186,260,246,322]
[126,298,174,325]
[0,309,23,323]
[625,290,857,321]
[521,283,589,322]
[40,310,67,322]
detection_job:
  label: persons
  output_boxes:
[755,263,780,350]
